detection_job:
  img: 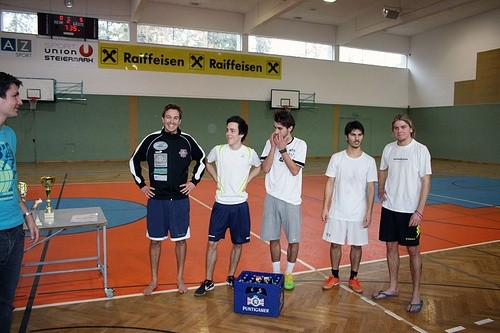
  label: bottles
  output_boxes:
[238,274,282,296]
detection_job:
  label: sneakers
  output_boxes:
[194,280,214,296]
[348,277,364,292]
[284,272,294,289]
[226,276,234,287]
[322,275,340,289]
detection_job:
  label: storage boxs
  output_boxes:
[233,270,285,317]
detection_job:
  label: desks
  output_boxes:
[20,206,114,299]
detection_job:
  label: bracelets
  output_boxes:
[279,148,286,154]
[22,212,33,219]
[415,210,423,219]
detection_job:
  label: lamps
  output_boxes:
[64,0,74,8]
[382,8,399,20]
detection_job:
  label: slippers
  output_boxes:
[406,300,423,312]
[372,290,398,299]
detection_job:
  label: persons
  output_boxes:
[372,113,432,314]
[260,111,308,289]
[0,72,39,333]
[194,114,262,296]
[129,103,205,295]
[321,120,378,291]
